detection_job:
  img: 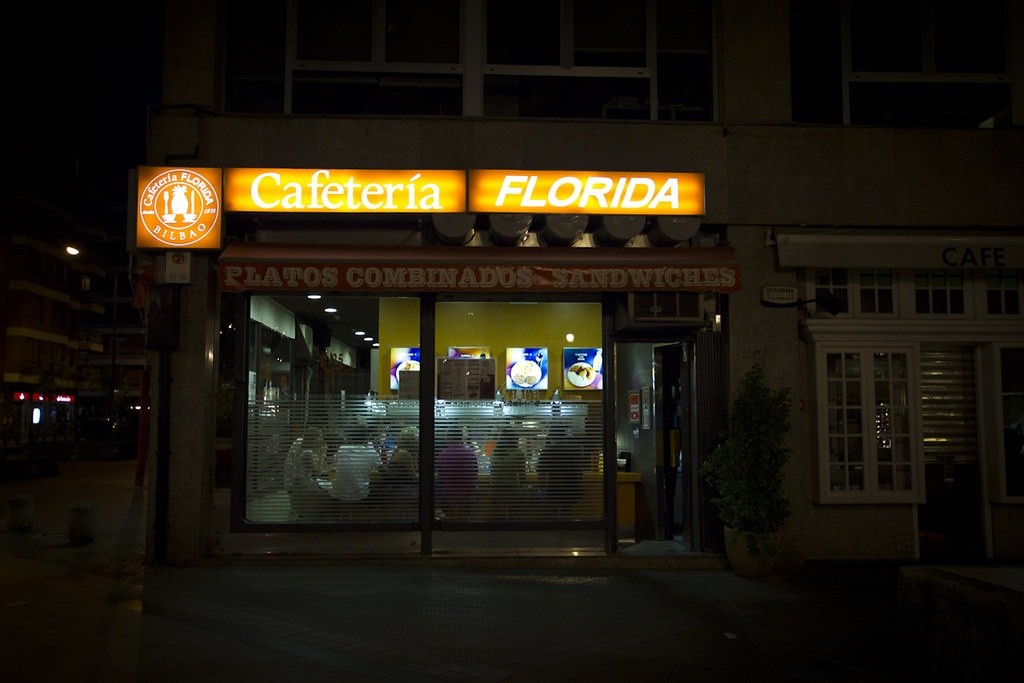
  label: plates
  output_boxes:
[568,363,596,387]
[511,360,542,388]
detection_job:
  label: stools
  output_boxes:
[288,510,299,521]
[543,502,571,521]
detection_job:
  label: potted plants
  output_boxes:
[696,348,796,583]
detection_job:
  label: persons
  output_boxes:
[536,423,584,522]
[437,423,479,518]
[489,425,530,521]
[281,418,420,523]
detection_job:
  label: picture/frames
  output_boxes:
[641,386,652,430]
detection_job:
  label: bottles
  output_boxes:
[593,349,602,372]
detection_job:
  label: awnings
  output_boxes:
[217,240,741,296]
